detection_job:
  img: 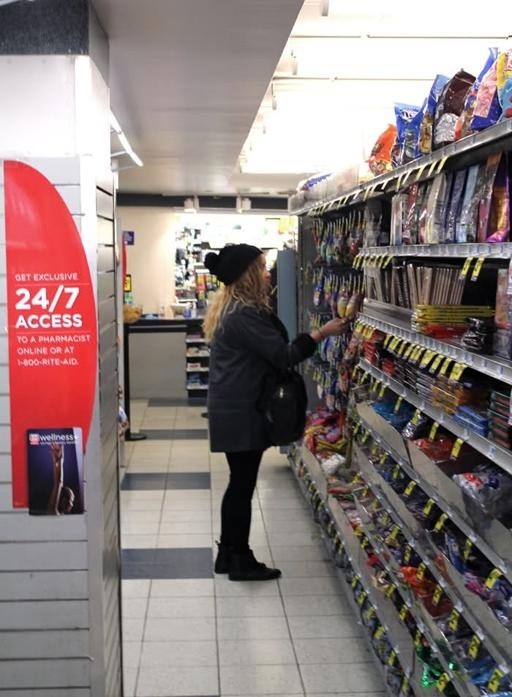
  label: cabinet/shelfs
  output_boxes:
[289,119,511,695]
[183,331,210,392]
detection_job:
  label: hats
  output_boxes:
[204,244,264,286]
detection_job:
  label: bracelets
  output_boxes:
[312,328,325,342]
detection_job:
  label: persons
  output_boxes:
[204,244,349,581]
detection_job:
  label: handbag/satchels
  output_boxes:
[263,342,308,447]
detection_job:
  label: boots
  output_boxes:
[214,542,282,581]
